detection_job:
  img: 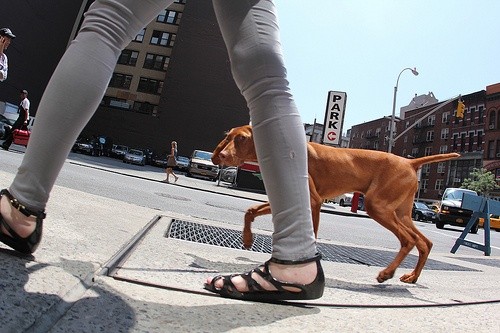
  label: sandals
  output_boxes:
[0,188,46,254]
[203,253,324,302]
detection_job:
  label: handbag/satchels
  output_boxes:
[11,124,31,145]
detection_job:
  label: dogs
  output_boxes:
[209,122,463,285]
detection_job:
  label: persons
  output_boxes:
[0,90,31,151]
[160,141,179,184]
[0,0,324,300]
[0,28,16,82]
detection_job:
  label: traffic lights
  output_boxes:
[456,101,466,119]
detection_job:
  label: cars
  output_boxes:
[27,116,36,132]
[72,132,129,161]
[174,156,190,173]
[144,149,238,185]
[123,148,147,166]
[323,193,500,234]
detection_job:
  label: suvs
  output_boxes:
[435,188,479,234]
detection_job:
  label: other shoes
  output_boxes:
[175,176,178,182]
[0,143,9,150]
[163,180,170,182]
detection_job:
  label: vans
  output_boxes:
[0,101,20,140]
[185,150,220,182]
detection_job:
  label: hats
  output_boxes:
[21,90,28,94]
[0,28,16,39]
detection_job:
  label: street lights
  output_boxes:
[388,67,419,153]
